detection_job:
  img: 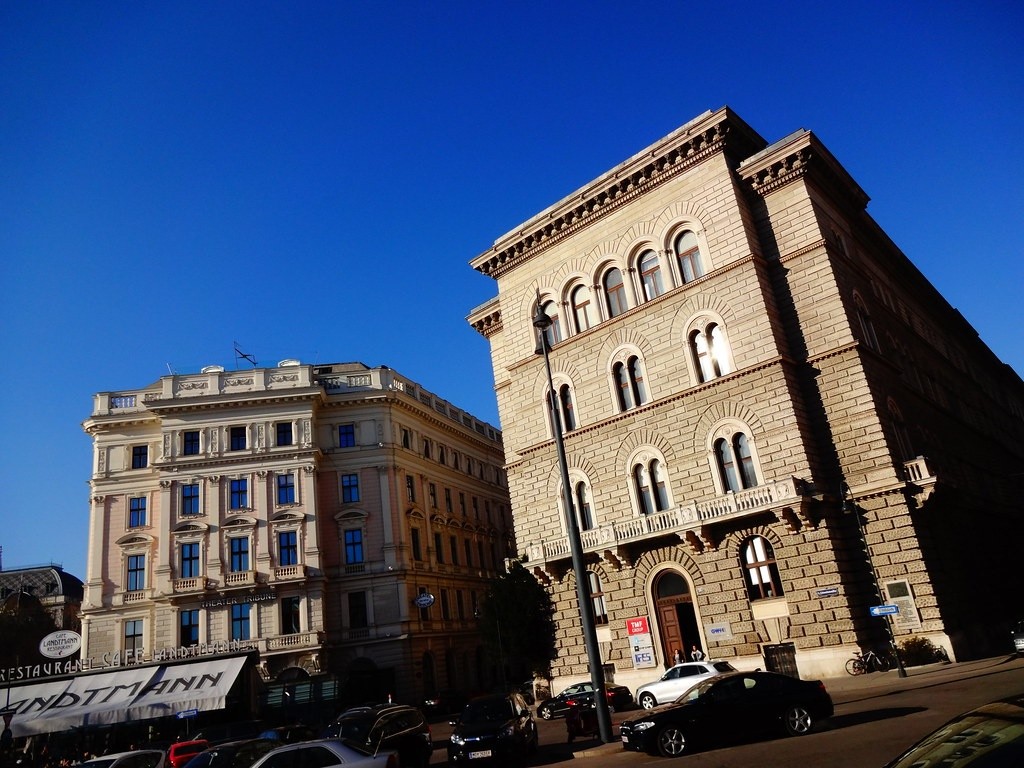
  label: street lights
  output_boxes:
[529,296,620,746]
[838,480,906,677]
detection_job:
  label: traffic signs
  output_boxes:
[869,604,899,616]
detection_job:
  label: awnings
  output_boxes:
[0,650,254,739]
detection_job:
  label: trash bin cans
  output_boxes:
[762,641,800,680]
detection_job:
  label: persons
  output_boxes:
[40,742,136,768]
[673,649,685,677]
[691,644,703,674]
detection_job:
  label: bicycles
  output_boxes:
[844,646,891,676]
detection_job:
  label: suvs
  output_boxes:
[314,702,435,768]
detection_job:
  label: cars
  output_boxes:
[535,681,632,719]
[633,660,740,712]
[445,691,539,768]
[76,722,401,768]
[881,692,1024,768]
[618,670,835,759]
